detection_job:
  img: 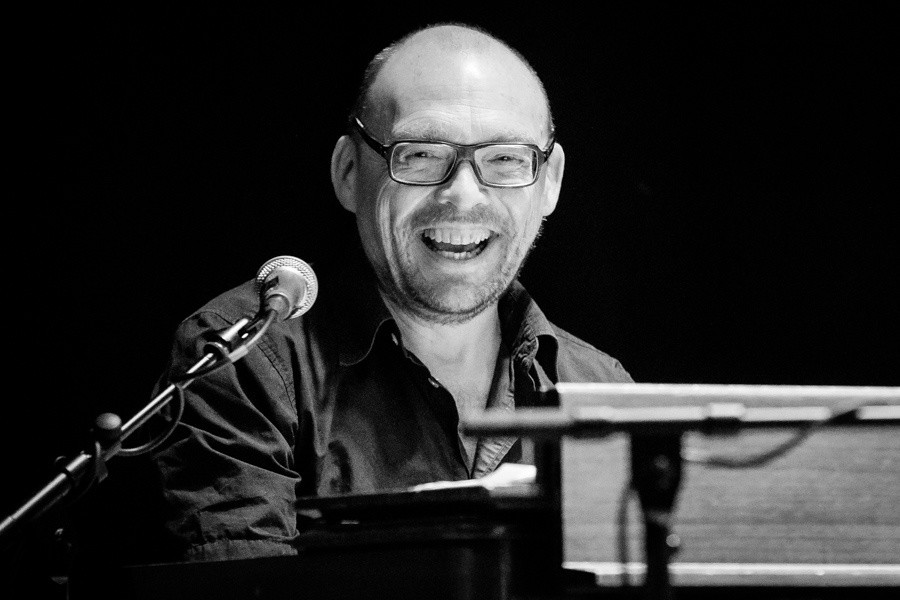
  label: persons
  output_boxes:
[137,25,634,567]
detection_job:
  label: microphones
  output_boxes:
[256,255,318,323]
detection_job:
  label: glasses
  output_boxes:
[351,115,555,189]
